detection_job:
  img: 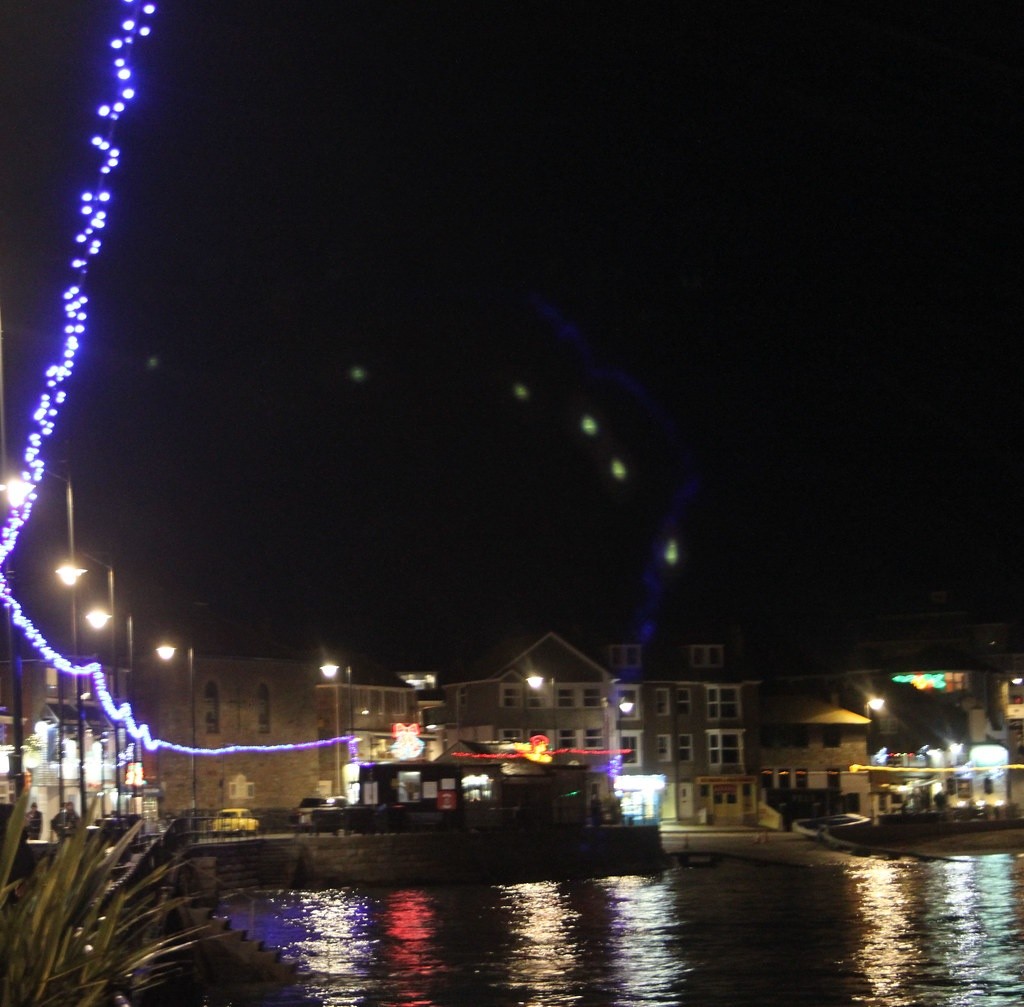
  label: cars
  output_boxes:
[212,809,259,837]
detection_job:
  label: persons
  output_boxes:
[26,802,43,841]
[934,790,948,810]
[50,801,80,840]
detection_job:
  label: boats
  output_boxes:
[790,808,952,843]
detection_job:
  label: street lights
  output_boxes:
[320,657,353,736]
[525,670,559,749]
[153,640,196,812]
[55,547,134,823]
